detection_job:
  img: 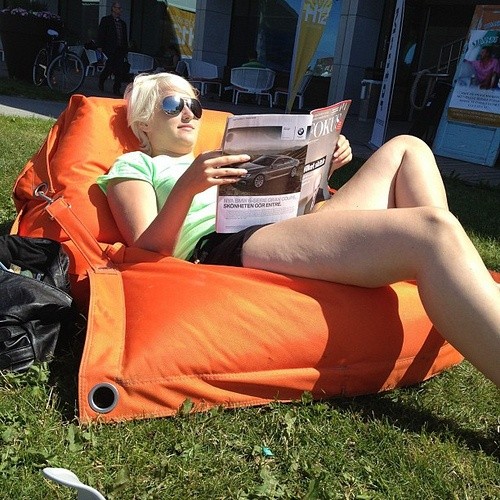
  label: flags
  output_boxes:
[288,0,333,111]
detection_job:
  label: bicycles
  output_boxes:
[32,28,86,95]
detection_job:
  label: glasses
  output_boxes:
[153,96,202,118]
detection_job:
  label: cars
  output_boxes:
[233,154,300,191]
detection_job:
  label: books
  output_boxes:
[214,98,353,233]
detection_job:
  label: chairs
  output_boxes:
[272,67,312,110]
[175,58,188,74]
[58,44,85,72]
[183,57,222,97]
[82,44,108,77]
[109,51,154,82]
[230,67,276,109]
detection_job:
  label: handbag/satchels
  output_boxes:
[0,234,87,385]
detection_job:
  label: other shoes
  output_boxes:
[99,80,105,91]
[114,89,122,96]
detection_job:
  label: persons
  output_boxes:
[94,1,130,98]
[242,49,268,69]
[96,72,500,399]
[467,46,500,90]
[456,47,479,87]
